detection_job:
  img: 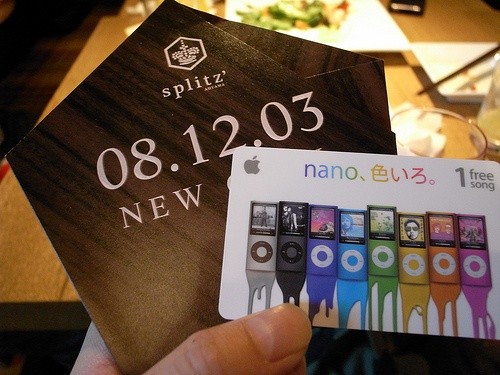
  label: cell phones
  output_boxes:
[388,0,425,15]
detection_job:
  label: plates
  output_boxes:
[225,0,411,51]
[410,40,499,103]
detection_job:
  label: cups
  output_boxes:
[479,57,500,151]
[390,108,488,159]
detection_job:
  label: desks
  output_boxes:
[0,1,500,331]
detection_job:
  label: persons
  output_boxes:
[70,302,313,375]
